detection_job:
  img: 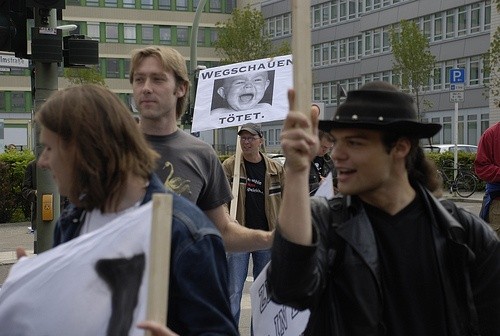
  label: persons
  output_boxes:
[8,144,17,150]
[22,158,37,255]
[16,84,240,336]
[474,120,500,223]
[265,79,500,336]
[130,45,276,336]
[211,70,272,115]
[309,130,339,201]
[222,123,285,336]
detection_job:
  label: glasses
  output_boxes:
[240,136,260,142]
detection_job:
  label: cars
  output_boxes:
[420,143,481,154]
[263,152,321,196]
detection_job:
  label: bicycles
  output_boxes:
[434,163,478,198]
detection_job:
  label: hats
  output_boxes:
[318,81,442,139]
[237,123,263,138]
